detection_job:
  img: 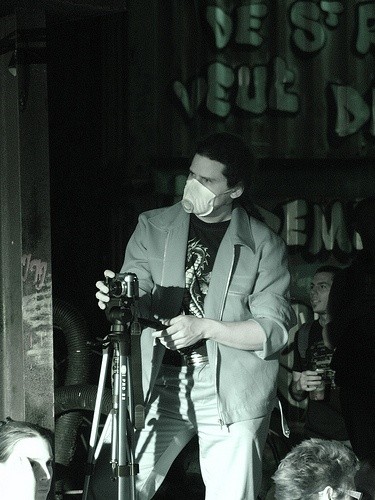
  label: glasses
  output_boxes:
[317,488,363,500]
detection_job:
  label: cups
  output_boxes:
[309,368,325,400]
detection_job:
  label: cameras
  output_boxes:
[105,272,139,298]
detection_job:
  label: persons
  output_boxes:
[91,133,298,500]
[288,265,349,440]
[270,437,360,500]
[326,197,374,464]
[0,421,54,500]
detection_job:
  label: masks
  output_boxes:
[181,178,233,216]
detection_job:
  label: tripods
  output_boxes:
[82,297,171,500]
